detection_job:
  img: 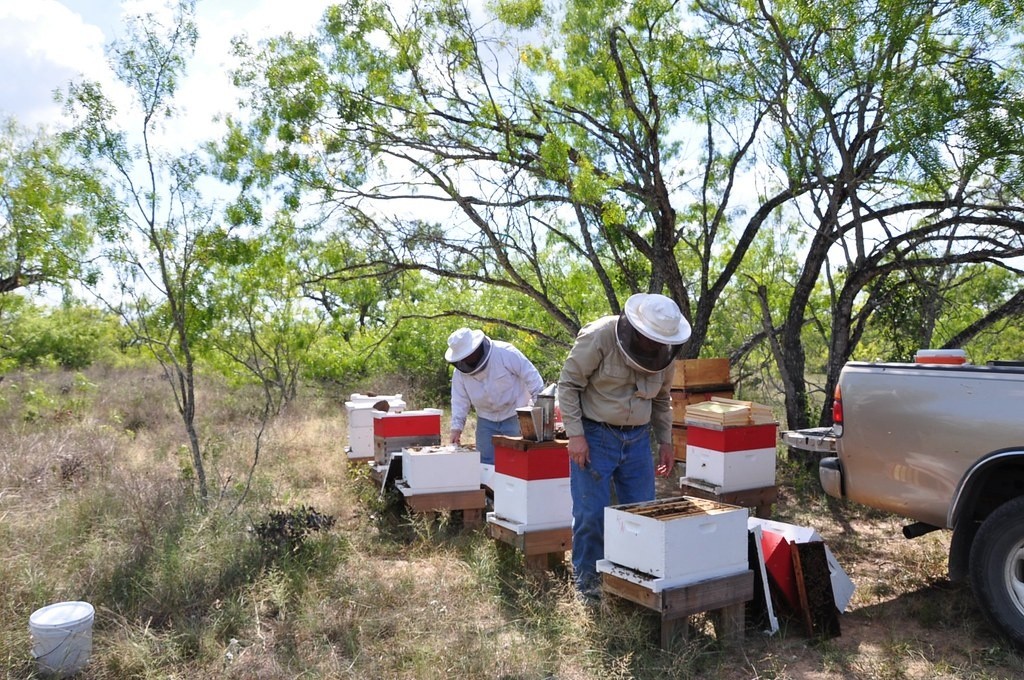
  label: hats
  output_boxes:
[615,293,692,374]
[444,327,492,375]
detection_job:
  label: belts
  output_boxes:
[588,418,646,431]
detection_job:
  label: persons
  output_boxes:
[444,327,544,466]
[557,293,691,608]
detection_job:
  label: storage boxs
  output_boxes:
[346,358,780,594]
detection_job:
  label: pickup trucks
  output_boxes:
[776,348,1024,647]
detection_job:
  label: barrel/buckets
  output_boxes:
[29,601,95,670]
[914,349,967,367]
